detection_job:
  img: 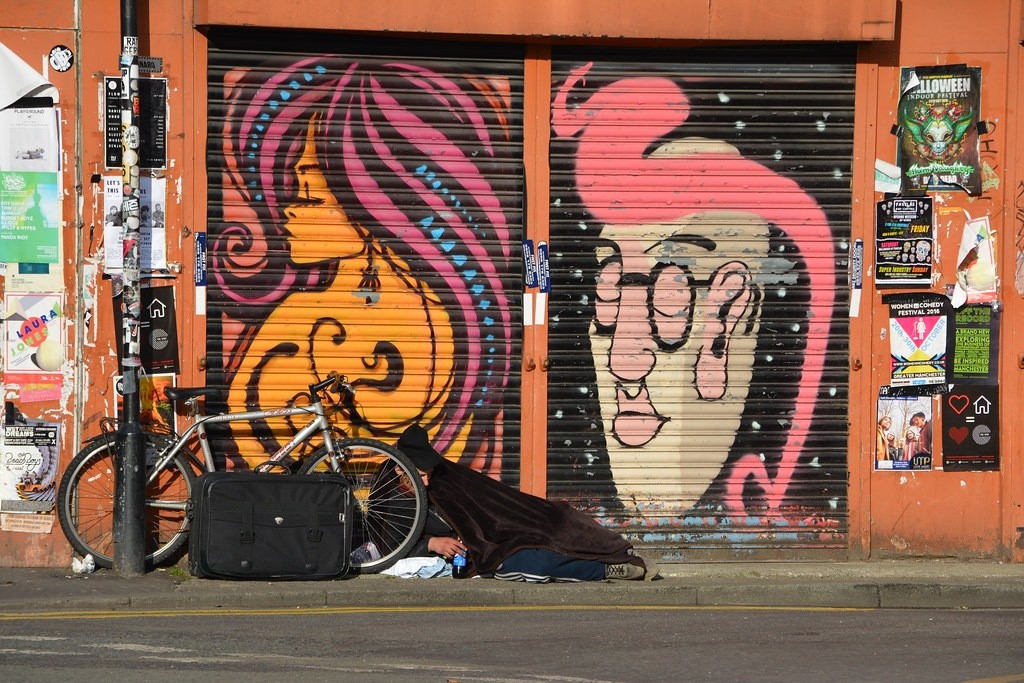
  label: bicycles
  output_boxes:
[58,371,429,575]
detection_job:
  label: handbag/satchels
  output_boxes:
[187,460,353,582]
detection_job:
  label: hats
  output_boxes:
[398,424,446,471]
[909,412,925,426]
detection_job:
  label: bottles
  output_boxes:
[452,540,468,578]
[350,542,381,574]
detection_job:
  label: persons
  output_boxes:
[140,202,165,228]
[105,203,123,227]
[877,412,932,461]
[366,425,643,582]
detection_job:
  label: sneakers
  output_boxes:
[604,562,645,580]
[627,548,660,581]
